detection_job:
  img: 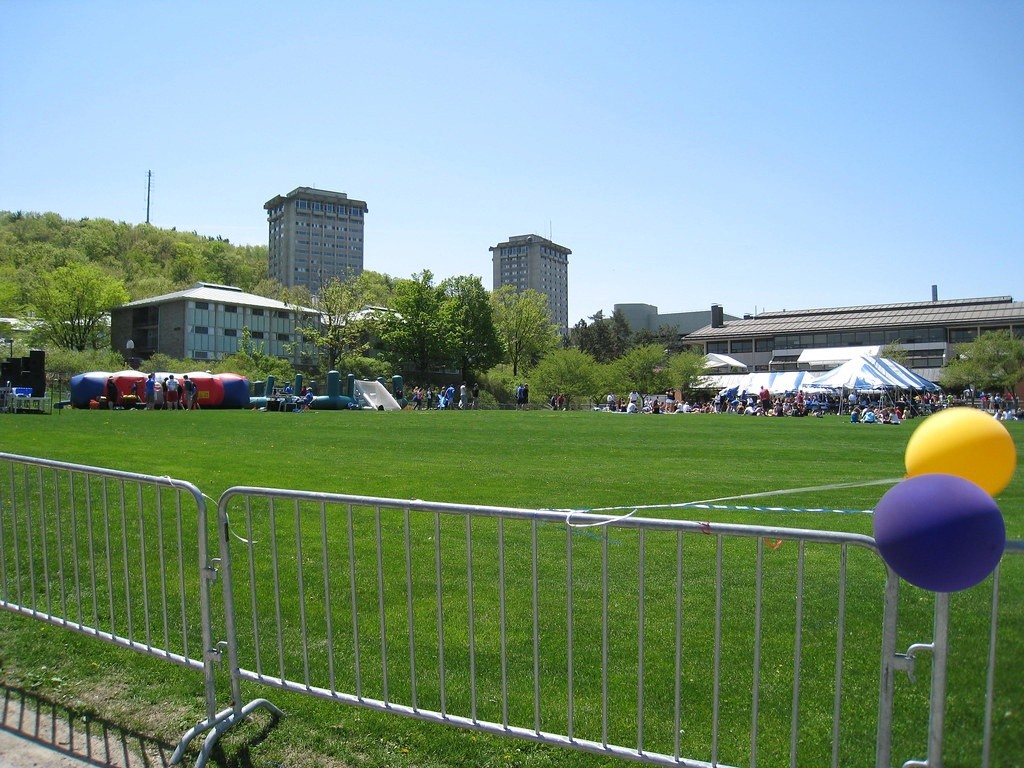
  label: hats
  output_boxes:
[685,402,688,405]
[306,387,313,390]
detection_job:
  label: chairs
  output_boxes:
[298,398,317,414]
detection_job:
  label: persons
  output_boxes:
[395,387,403,407]
[850,406,911,425]
[713,391,839,418]
[664,386,675,414]
[130,372,201,410]
[643,396,714,414]
[914,391,953,414]
[105,375,118,409]
[551,394,567,411]
[979,392,1024,421]
[604,390,640,414]
[759,385,770,416]
[517,384,529,412]
[286,384,313,411]
[413,383,479,410]
[843,391,878,415]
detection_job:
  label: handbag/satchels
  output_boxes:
[413,395,417,401]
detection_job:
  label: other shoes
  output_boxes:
[460,406,463,410]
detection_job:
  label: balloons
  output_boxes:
[905,406,1016,496]
[872,473,1007,594]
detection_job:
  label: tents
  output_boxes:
[801,354,941,416]
[736,370,816,397]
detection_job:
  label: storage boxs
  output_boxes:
[12,388,33,397]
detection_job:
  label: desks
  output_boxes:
[278,403,297,412]
